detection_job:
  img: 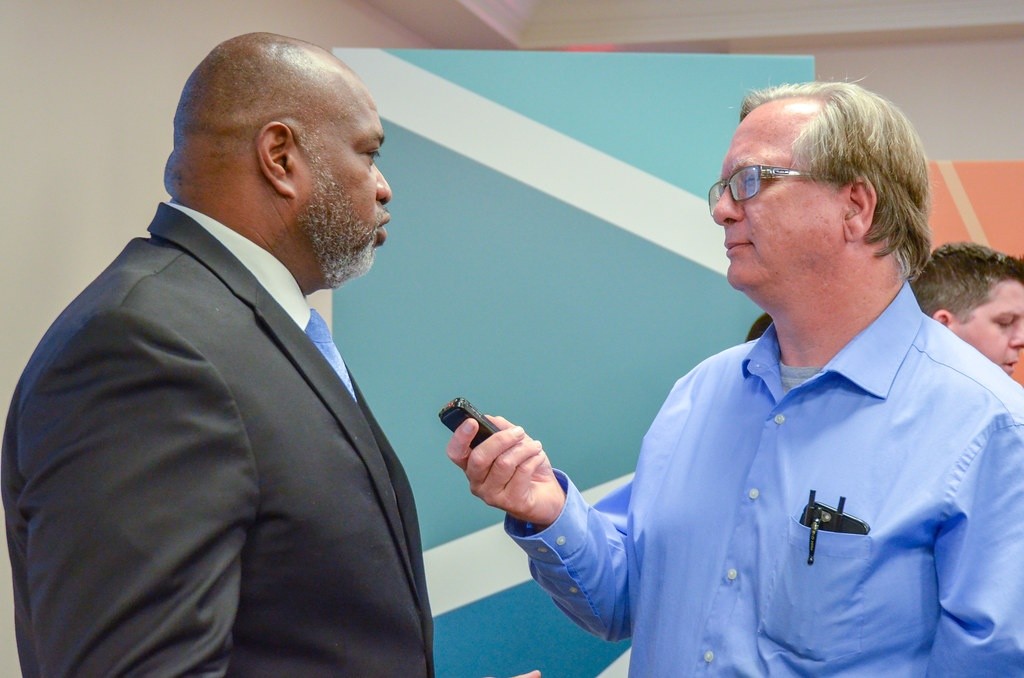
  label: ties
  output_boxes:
[306,309,358,403]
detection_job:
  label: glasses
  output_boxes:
[708,167,834,217]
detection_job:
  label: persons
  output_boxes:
[446,81,1024,678]
[0,31,437,678]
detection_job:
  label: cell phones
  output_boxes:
[798,502,870,534]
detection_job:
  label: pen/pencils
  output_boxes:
[808,505,821,565]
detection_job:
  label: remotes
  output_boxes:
[439,398,503,450]
